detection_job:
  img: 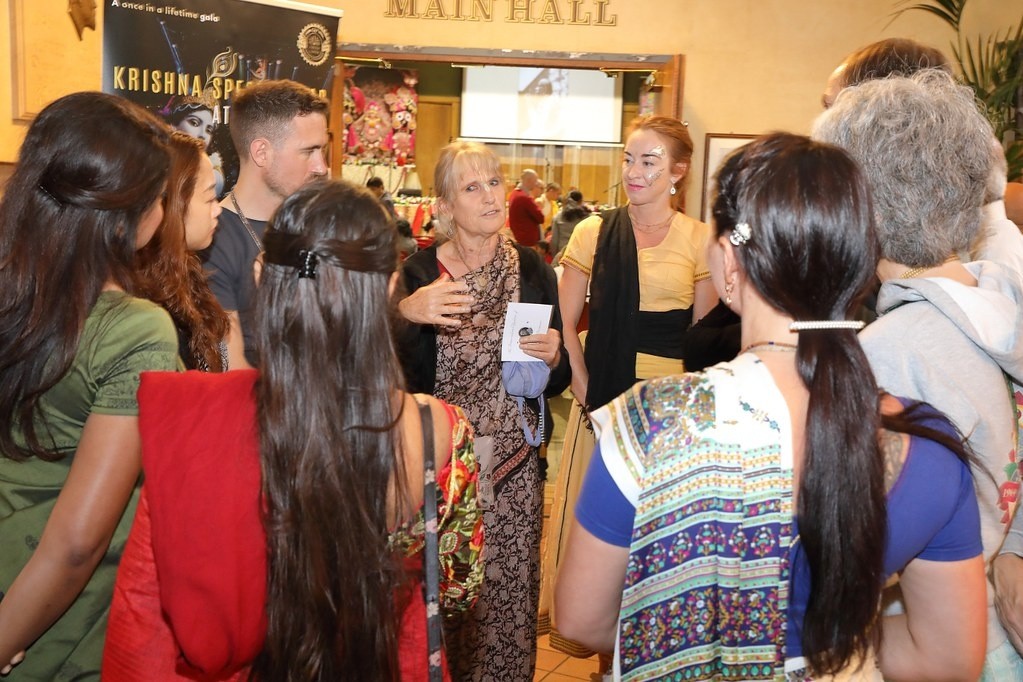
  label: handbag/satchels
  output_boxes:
[502,359,550,447]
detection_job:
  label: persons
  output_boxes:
[508,168,594,267]
[0,79,331,681]
[164,87,220,151]
[102,179,485,682]
[388,138,572,682]
[366,176,418,255]
[558,36,1023,682]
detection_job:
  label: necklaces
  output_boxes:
[629,212,676,234]
[455,246,503,337]
[738,341,798,355]
[230,191,264,253]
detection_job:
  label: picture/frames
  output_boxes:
[698,133,760,224]
